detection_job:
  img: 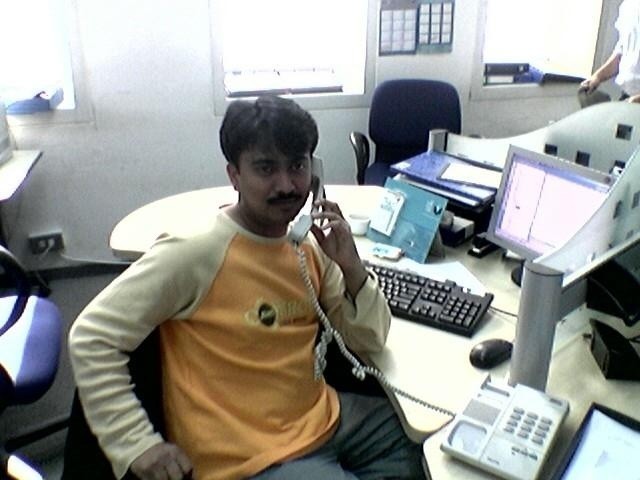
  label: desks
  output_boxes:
[0,148,55,300]
[412,308,640,479]
[106,167,608,447]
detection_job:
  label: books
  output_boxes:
[390,147,504,207]
[486,75,514,85]
[486,62,530,74]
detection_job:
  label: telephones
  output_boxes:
[285,155,324,247]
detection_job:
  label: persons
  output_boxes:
[69,93,427,480]
[578,0,639,106]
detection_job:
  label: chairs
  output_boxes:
[54,320,406,480]
[1,242,75,480]
[346,77,482,191]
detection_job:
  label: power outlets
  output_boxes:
[24,229,68,256]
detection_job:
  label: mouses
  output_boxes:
[468,340,513,370]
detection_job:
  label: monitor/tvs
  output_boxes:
[484,143,619,291]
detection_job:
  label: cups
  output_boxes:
[347,213,371,236]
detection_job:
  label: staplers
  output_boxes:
[467,237,501,258]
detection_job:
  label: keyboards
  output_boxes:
[364,263,494,338]
